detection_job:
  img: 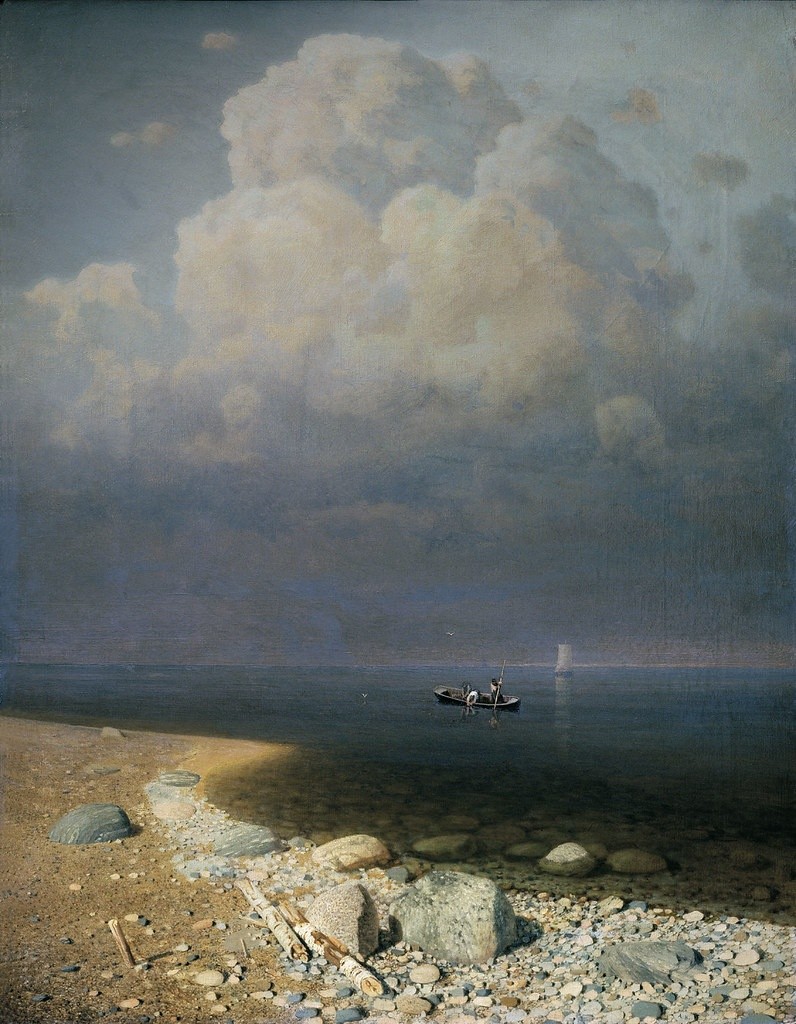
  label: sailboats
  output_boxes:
[554,644,573,678]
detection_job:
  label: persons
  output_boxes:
[489,709,503,729]
[460,682,479,705]
[462,706,475,721]
[490,679,505,703]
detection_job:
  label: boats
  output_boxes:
[431,685,522,712]
